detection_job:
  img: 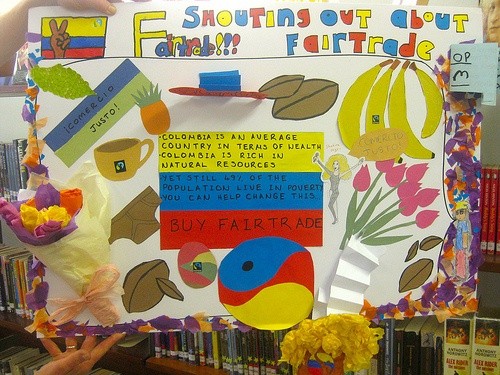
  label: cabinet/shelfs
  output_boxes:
[0,68,500,374]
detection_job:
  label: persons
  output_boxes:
[487,0,500,42]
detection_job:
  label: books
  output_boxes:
[153,317,292,375]
[480,165,500,256]
[372,294,500,375]
[0,136,29,205]
[0,243,35,320]
[0,330,122,375]
